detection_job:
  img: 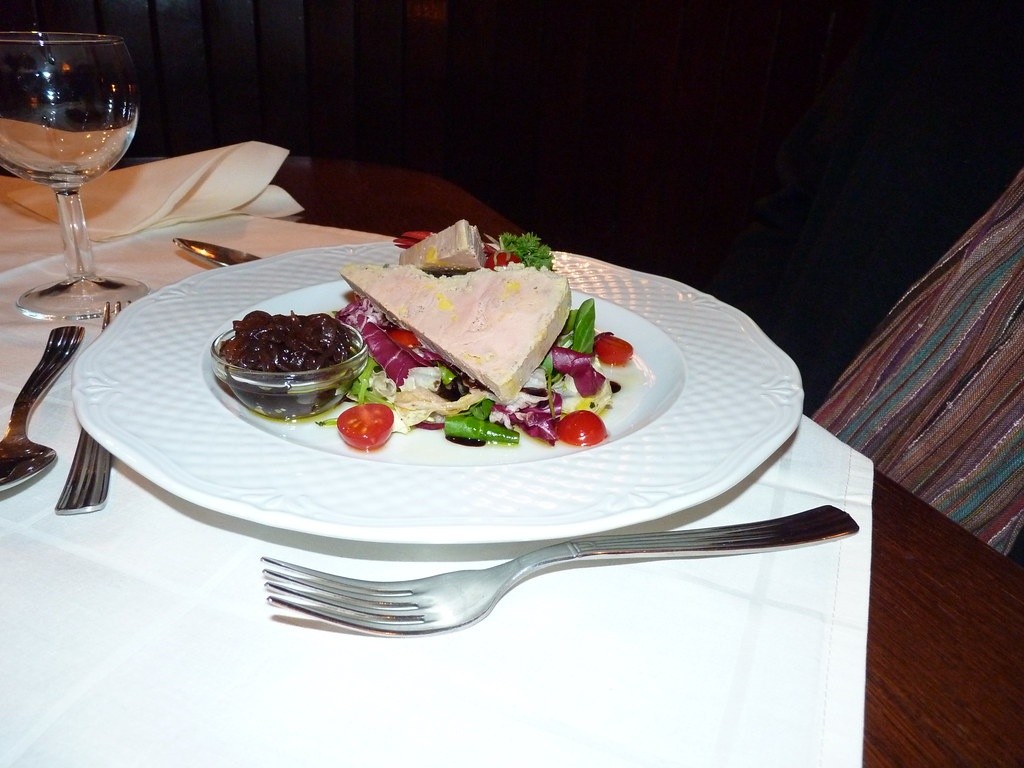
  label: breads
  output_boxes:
[338,262,572,401]
[398,220,486,272]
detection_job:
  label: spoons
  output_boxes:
[173,237,261,268]
[0,327,85,491]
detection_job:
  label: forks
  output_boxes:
[54,297,136,517]
[261,503,859,634]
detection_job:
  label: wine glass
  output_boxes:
[1,30,148,321]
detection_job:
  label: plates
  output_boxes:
[71,243,805,547]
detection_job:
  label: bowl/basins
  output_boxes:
[212,327,367,419]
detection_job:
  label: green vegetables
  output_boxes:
[496,230,556,274]
[561,299,597,356]
[334,357,519,445]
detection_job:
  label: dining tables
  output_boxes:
[0,158,1021,767]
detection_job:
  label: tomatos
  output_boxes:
[594,332,634,366]
[338,402,394,450]
[554,408,609,448]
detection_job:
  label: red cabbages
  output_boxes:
[486,347,606,446]
[333,293,436,390]
